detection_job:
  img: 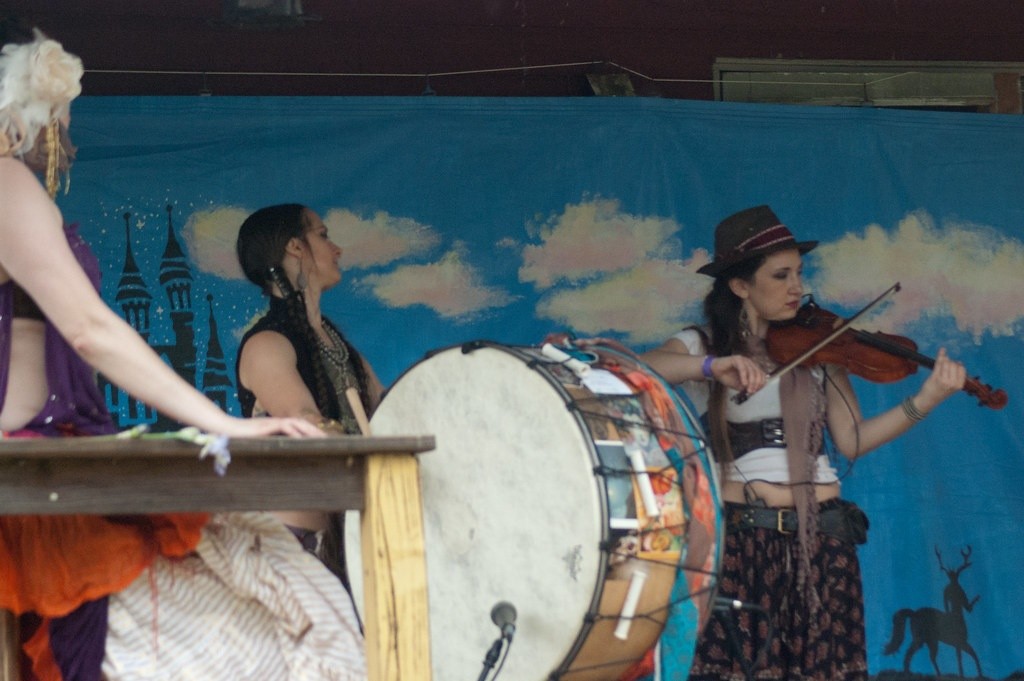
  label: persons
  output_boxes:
[235,204,387,630]
[0,27,368,681]
[638,206,967,681]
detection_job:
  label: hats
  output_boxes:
[695,203,823,278]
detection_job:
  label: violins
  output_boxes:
[765,304,1009,410]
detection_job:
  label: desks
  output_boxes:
[0,433,437,681]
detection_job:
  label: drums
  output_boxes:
[337,333,689,681]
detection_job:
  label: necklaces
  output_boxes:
[312,318,348,364]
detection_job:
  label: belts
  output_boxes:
[715,505,851,542]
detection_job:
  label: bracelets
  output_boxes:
[901,396,926,422]
[703,354,716,380]
